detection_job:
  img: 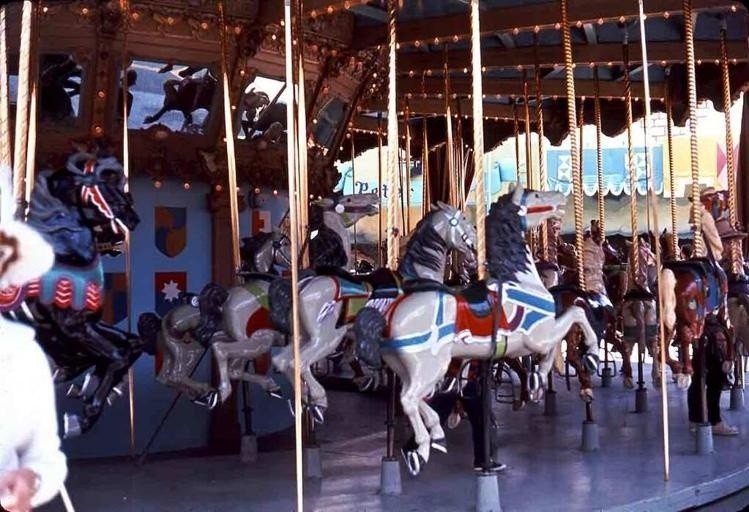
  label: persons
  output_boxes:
[690,329,737,435]
[680,243,693,262]
[400,373,507,473]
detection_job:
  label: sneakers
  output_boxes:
[401,448,413,470]
[712,421,738,435]
[473,460,505,470]
